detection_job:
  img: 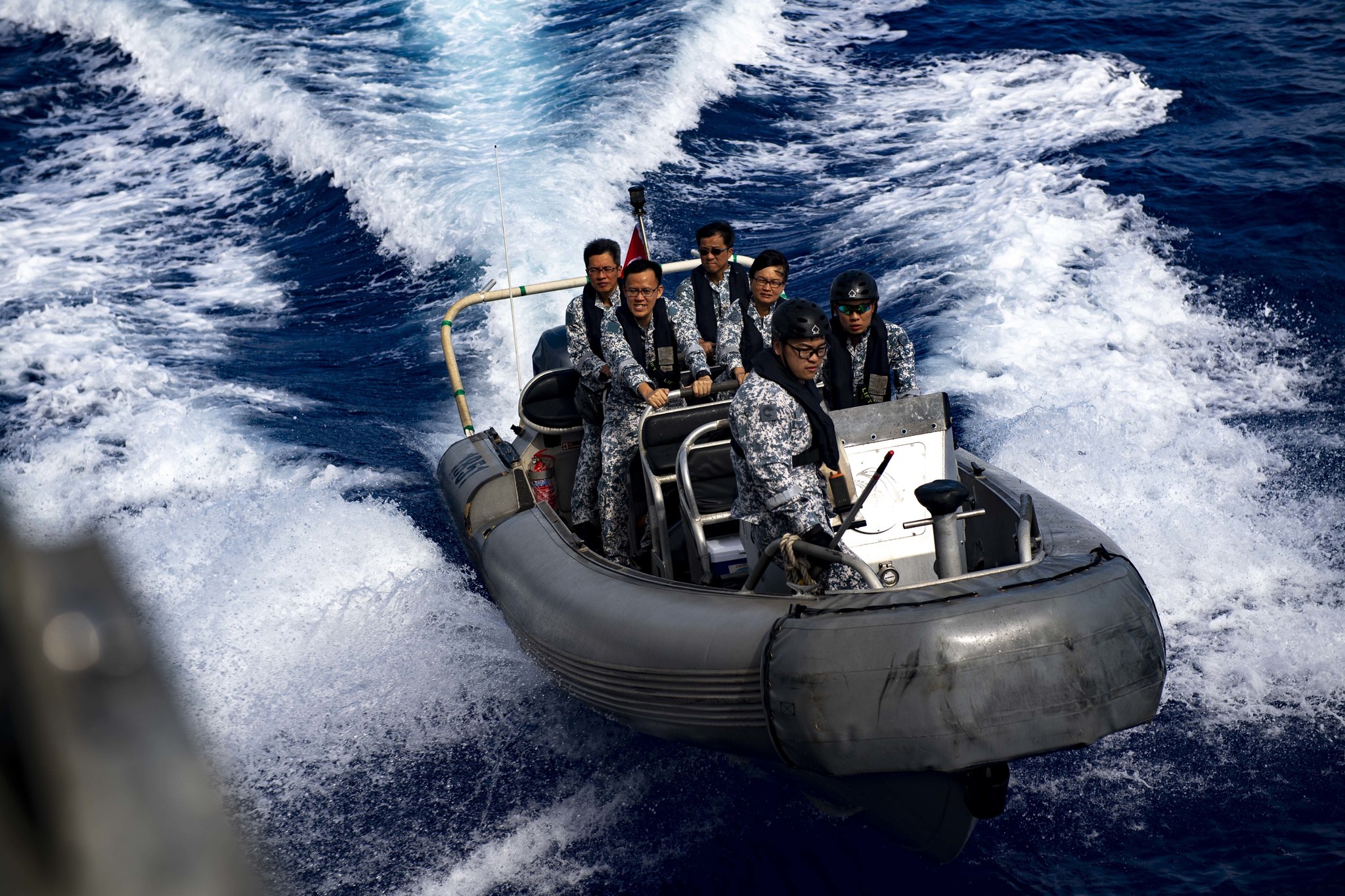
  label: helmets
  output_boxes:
[828,269,880,302]
[768,297,832,343]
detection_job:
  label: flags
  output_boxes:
[620,221,647,280]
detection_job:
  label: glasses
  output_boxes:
[585,266,618,274]
[625,286,659,297]
[836,302,873,316]
[787,340,832,360]
[697,248,729,256]
[753,274,784,288]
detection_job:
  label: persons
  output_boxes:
[727,297,869,592]
[599,259,713,569]
[565,237,627,552]
[707,249,790,401]
[674,219,751,365]
[814,269,921,411]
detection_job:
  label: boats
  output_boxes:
[431,248,1170,869]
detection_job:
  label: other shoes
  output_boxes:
[573,520,594,547]
[632,544,653,556]
[621,558,644,573]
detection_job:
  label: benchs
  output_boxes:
[519,363,825,589]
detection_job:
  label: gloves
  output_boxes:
[802,523,842,551]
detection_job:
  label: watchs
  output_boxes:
[695,369,711,380]
